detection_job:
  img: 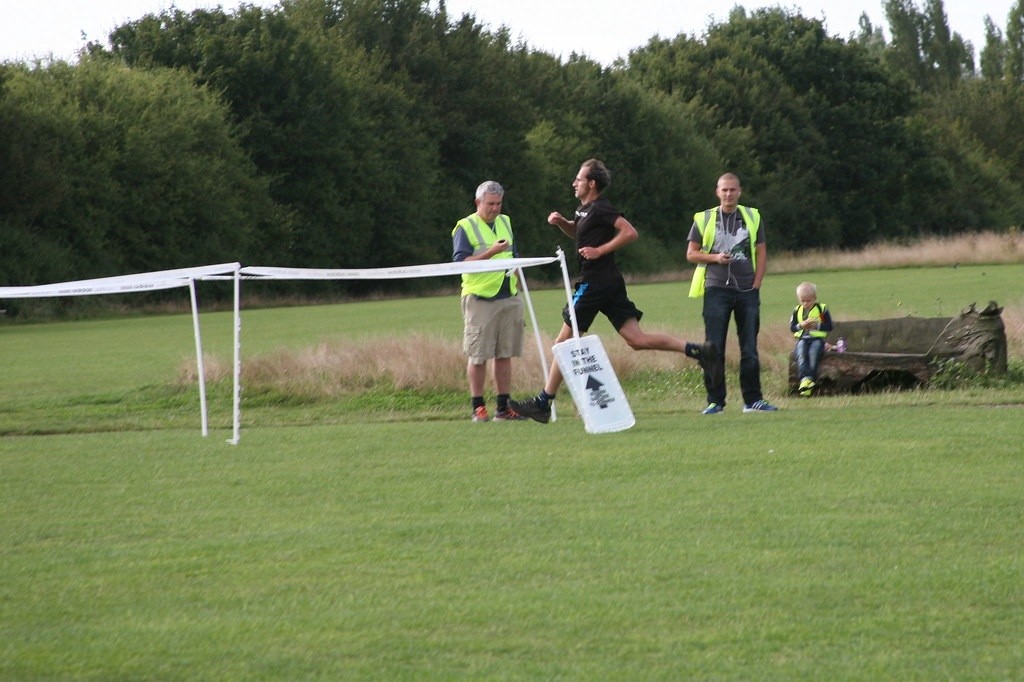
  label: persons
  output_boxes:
[684,171,780,416]
[788,279,834,398]
[450,179,528,423]
[507,157,723,427]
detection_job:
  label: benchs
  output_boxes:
[788,299,1008,399]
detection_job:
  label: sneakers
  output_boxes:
[702,403,724,415]
[471,406,490,422]
[698,341,721,388]
[797,379,816,396]
[742,399,778,412]
[491,407,529,421]
[507,398,552,424]
